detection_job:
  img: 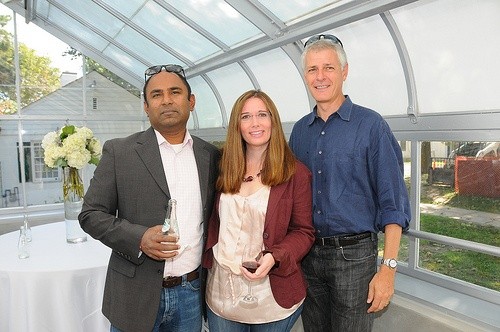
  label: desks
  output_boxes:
[0,220,113,332]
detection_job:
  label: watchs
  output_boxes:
[381,258,398,268]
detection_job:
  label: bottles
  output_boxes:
[161,200,180,261]
[18,226,28,259]
[22,214,31,242]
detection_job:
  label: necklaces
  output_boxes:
[242,170,262,182]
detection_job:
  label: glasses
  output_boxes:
[304,34,343,48]
[144,64,186,83]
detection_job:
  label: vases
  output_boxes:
[63,167,88,244]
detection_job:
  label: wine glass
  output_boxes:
[240,244,263,304]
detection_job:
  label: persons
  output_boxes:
[201,90,315,332]
[288,39,411,331]
[77,69,220,332]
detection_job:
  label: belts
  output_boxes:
[162,271,199,288]
[316,233,371,246]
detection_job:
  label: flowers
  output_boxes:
[40,117,103,200]
